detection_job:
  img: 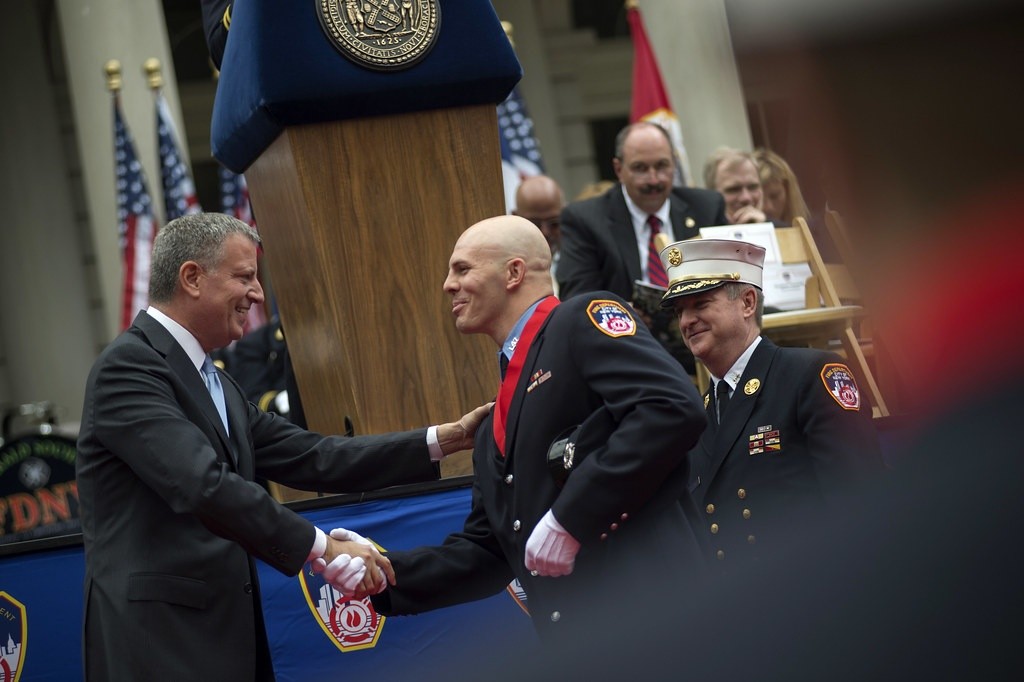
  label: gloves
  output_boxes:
[525,508,582,577]
[311,528,388,593]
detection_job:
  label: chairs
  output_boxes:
[656,216,888,418]
[818,208,892,401]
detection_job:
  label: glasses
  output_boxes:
[529,215,560,230]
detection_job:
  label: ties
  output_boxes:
[202,354,229,438]
[717,380,731,424]
[648,216,669,287]
[500,352,509,382]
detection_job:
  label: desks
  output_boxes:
[0,476,544,682]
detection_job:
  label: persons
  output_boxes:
[308,214,719,671]
[659,237,882,587]
[80,212,497,681]
[512,119,810,379]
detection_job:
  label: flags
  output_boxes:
[221,148,259,227]
[498,91,542,216]
[630,7,692,188]
[156,110,201,219]
[111,96,158,328]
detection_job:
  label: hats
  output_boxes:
[658,238,766,308]
